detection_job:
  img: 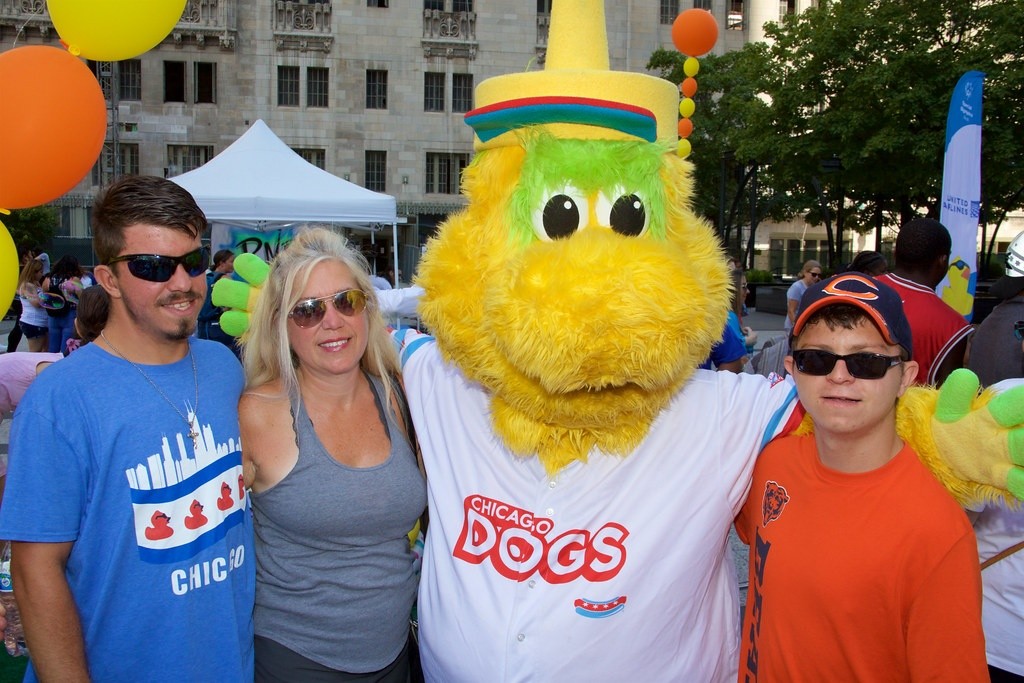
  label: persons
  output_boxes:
[0,174,1023,683]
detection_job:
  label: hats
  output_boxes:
[1005,231,1024,278]
[788,271,912,361]
[211,250,234,271]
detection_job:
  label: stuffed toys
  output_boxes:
[209,0,1023,683]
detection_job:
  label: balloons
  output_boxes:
[46,0,187,61]
[0,209,19,321]
[672,9,718,158]
[0,45,108,209]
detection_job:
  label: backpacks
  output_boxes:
[46,277,71,319]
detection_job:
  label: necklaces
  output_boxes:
[102,325,202,445]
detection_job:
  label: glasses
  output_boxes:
[793,349,905,379]
[106,245,210,283]
[1014,320,1024,341]
[742,283,748,288]
[809,272,823,278]
[288,289,370,329]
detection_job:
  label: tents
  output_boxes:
[166,118,400,334]
